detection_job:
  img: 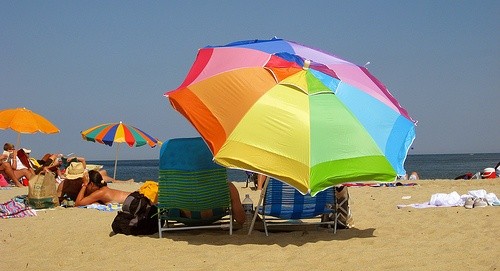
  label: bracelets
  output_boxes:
[13,159,16,160]
[82,184,87,188]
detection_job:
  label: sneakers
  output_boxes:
[465,197,475,209]
[473,198,487,208]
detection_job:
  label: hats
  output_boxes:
[65,162,88,180]
[22,148,31,153]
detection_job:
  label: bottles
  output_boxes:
[241,193,253,232]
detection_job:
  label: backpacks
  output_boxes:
[111,191,165,235]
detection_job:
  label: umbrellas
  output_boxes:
[163,37,418,235]
[0,108,60,146]
[80,122,157,179]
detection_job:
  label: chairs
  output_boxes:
[256,177,338,237]
[157,137,232,237]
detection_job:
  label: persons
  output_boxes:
[402,171,420,180]
[75,170,159,206]
[228,182,246,224]
[0,143,113,202]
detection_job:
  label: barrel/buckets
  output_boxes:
[481,167,497,179]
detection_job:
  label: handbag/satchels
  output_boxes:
[29,167,60,209]
